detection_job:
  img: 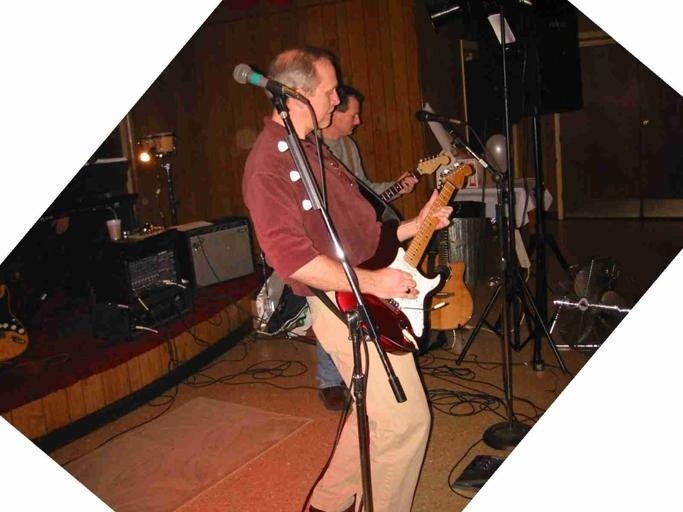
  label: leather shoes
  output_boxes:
[319,386,350,411]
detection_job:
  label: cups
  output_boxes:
[106,219,122,241]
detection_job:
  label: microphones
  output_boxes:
[417,110,467,129]
[233,64,309,105]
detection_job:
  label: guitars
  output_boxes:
[0,285,29,361]
[334,163,474,355]
[422,173,474,332]
[377,154,450,204]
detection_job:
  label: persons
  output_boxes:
[240,43,455,511]
[312,82,418,414]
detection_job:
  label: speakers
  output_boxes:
[413,0,521,131]
[521,0,585,117]
[171,216,256,290]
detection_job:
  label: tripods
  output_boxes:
[494,102,602,369]
[453,175,570,376]
